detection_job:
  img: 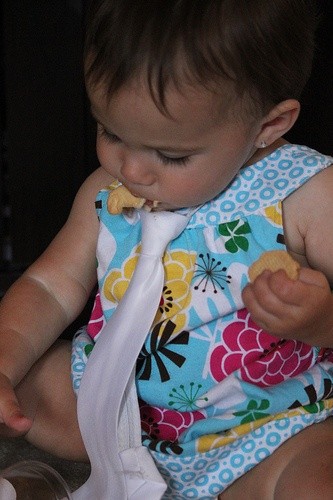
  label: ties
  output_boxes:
[71,202,207,500]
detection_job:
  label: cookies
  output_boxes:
[248,250,301,283]
[106,185,145,214]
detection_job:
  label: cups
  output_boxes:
[1,460,74,500]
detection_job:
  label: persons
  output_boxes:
[0,0,332,500]
